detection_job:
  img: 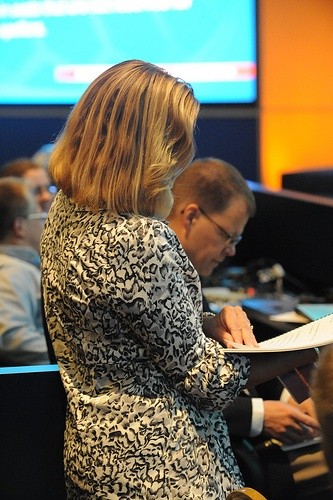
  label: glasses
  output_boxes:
[32,184,58,196]
[180,205,242,248]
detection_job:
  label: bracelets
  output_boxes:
[203,312,217,317]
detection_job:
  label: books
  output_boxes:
[219,312,333,353]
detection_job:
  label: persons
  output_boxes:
[41,156,320,500]
[0,176,48,352]
[0,159,51,213]
[39,60,260,500]
